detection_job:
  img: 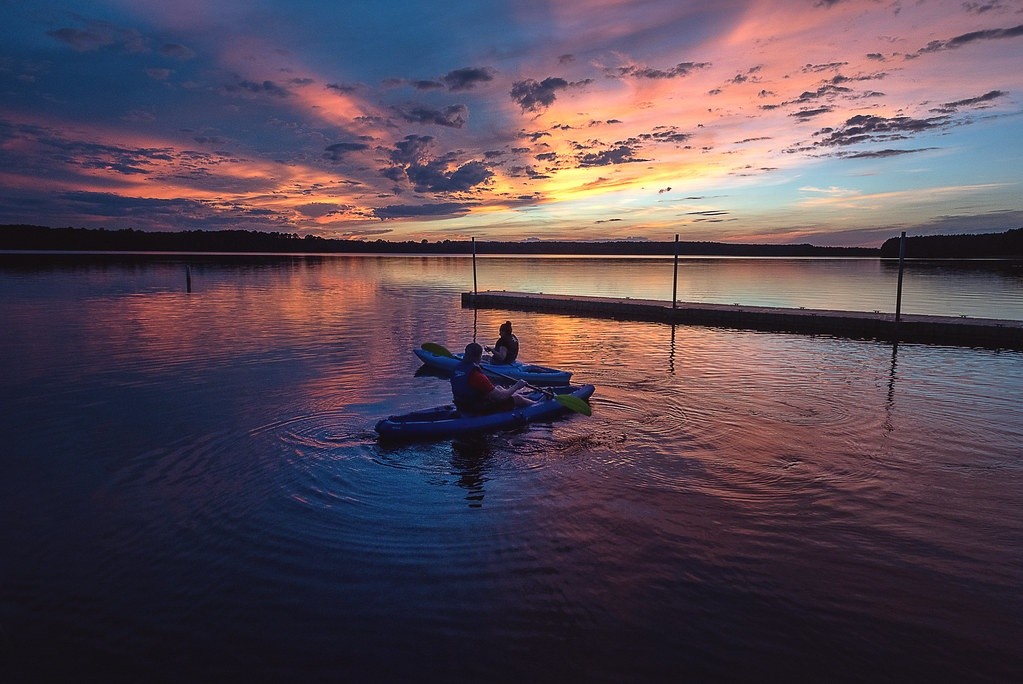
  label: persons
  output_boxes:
[482,321,519,365]
[450,343,554,416]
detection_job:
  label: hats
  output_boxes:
[465,343,482,361]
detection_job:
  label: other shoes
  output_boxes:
[539,388,554,402]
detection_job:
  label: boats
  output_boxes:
[413,348,573,387]
[373,383,595,437]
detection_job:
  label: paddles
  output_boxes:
[421,342,592,417]
